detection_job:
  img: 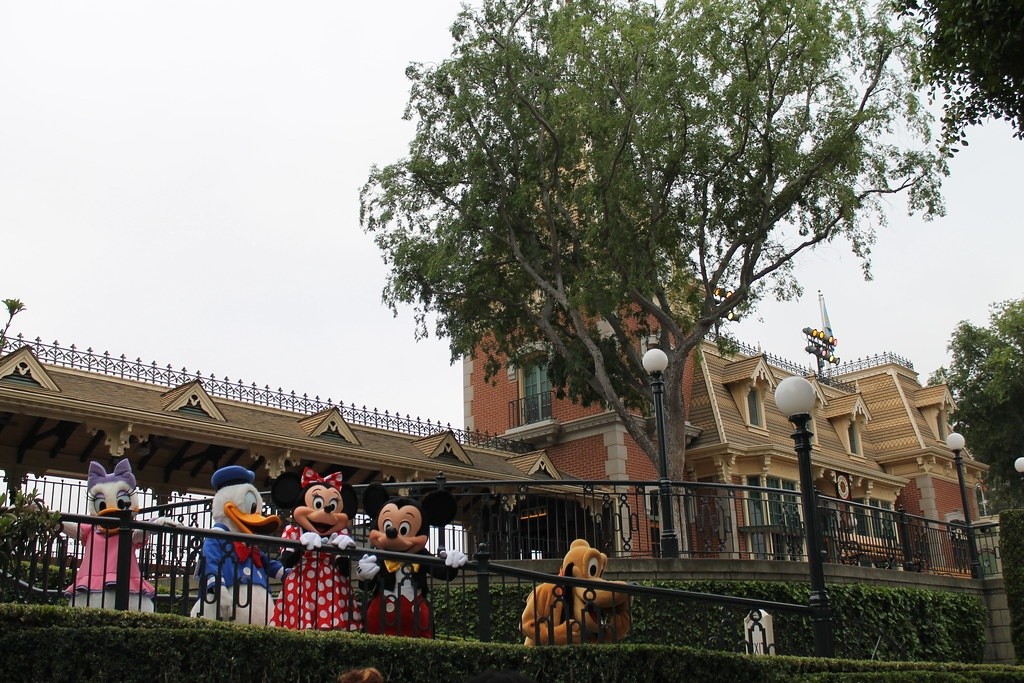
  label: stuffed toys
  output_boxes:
[190,466,294,627]
[52,459,183,613]
[519,539,635,647]
[357,482,469,639]
[269,466,362,631]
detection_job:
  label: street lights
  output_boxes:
[643,346,684,563]
[775,377,836,658]
[947,433,983,576]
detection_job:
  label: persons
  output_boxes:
[339,667,383,683]
